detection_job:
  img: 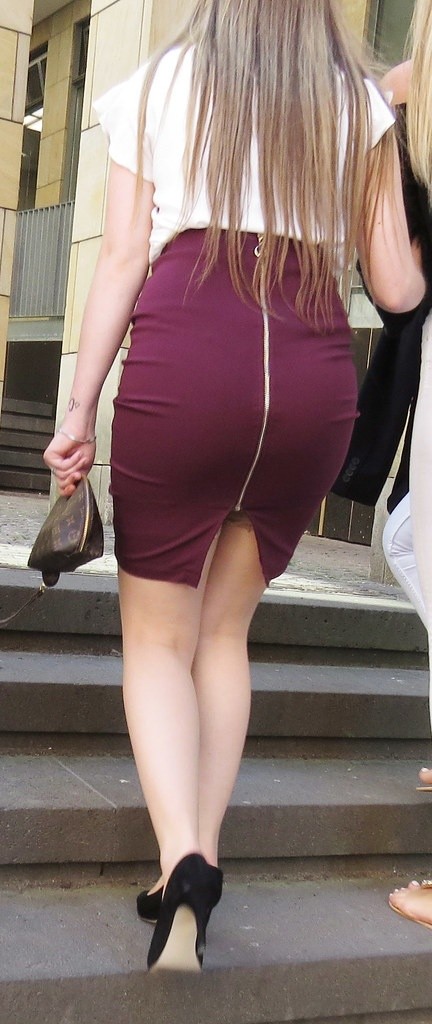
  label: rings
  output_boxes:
[51,468,55,473]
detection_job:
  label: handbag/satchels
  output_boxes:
[0,475,104,624]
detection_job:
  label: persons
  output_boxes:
[331,0,432,929]
[43,0,425,973]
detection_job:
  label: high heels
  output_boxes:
[147,853,210,971]
[136,864,223,923]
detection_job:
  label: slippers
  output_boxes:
[388,879,432,928]
[416,787,432,792]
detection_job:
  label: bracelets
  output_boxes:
[59,426,97,444]
